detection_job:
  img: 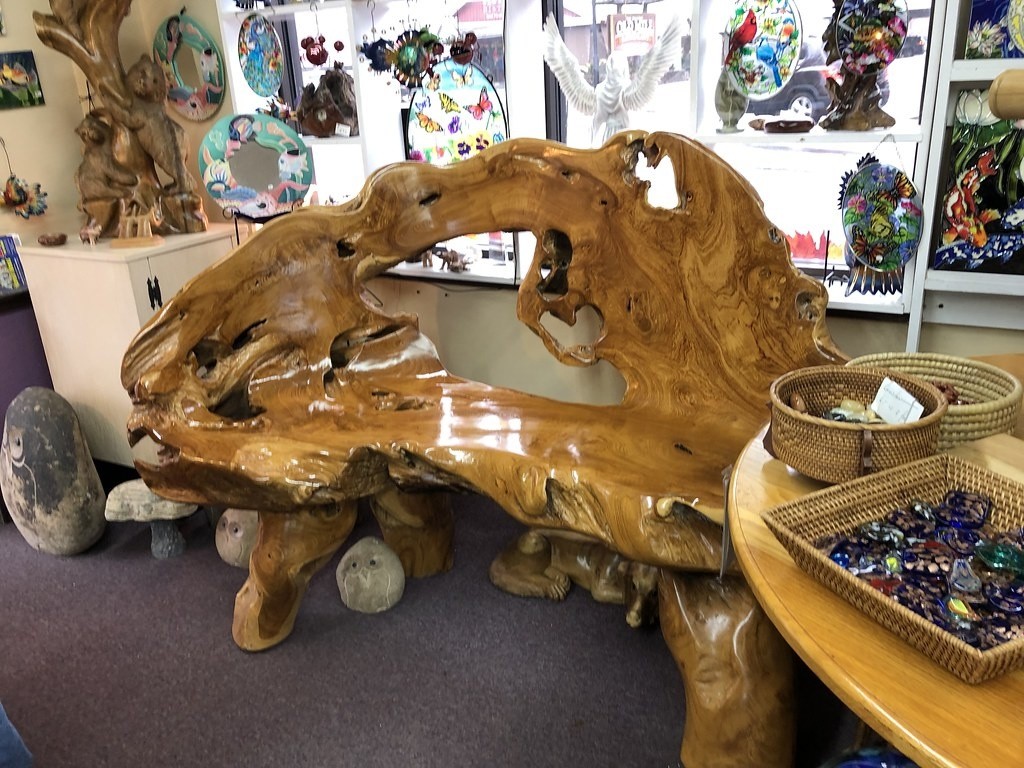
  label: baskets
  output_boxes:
[759,455,1024,683]
[844,353,1021,452]
[770,365,947,485]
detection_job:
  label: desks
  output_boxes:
[727,353,1024,768]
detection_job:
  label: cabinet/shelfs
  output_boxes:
[905,0,1024,353]
[15,222,251,485]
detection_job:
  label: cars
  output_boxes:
[745,35,890,124]
